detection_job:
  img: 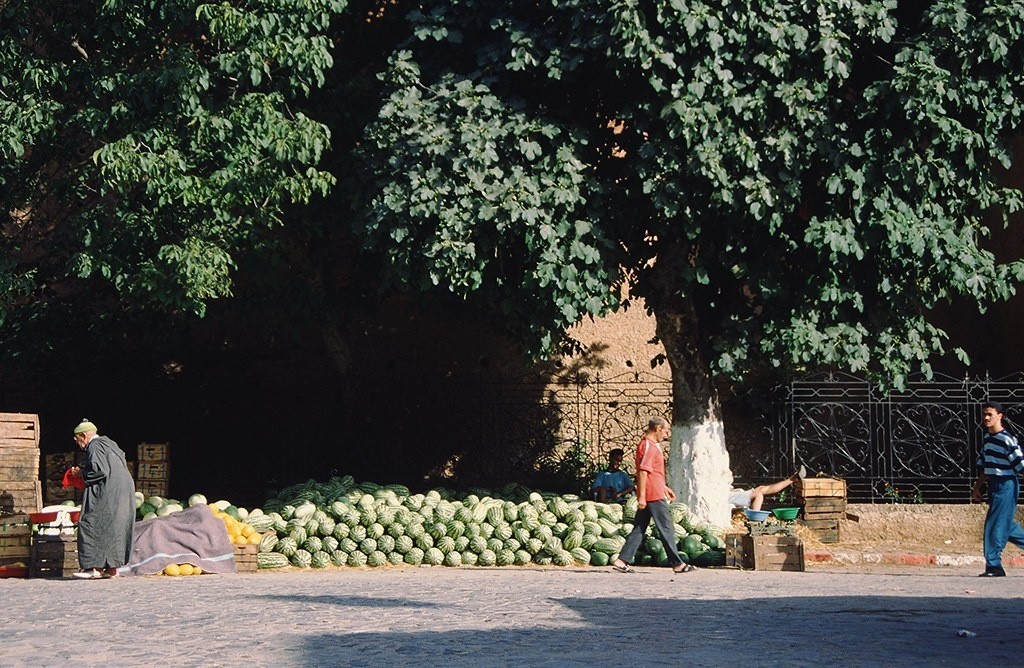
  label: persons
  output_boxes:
[71,418,135,579]
[728,464,806,511]
[613,416,697,574]
[592,448,634,505]
[973,402,1024,577]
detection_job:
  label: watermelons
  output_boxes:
[60,500,75,507]
[246,475,728,570]
[134,491,249,524]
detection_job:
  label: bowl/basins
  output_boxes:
[744,509,772,522]
[772,507,801,521]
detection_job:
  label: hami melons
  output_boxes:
[157,504,262,576]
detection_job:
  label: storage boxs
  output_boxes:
[0,412,173,578]
[725,475,847,573]
[232,543,258,574]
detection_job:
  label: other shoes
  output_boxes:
[979,566,1006,577]
[101,571,112,578]
[73,567,103,578]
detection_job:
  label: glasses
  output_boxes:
[74,433,83,440]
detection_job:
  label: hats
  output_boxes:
[74,418,97,433]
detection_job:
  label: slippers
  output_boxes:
[795,464,807,480]
[613,565,635,573]
[675,564,698,573]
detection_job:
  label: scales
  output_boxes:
[27,511,80,542]
[742,507,801,535]
